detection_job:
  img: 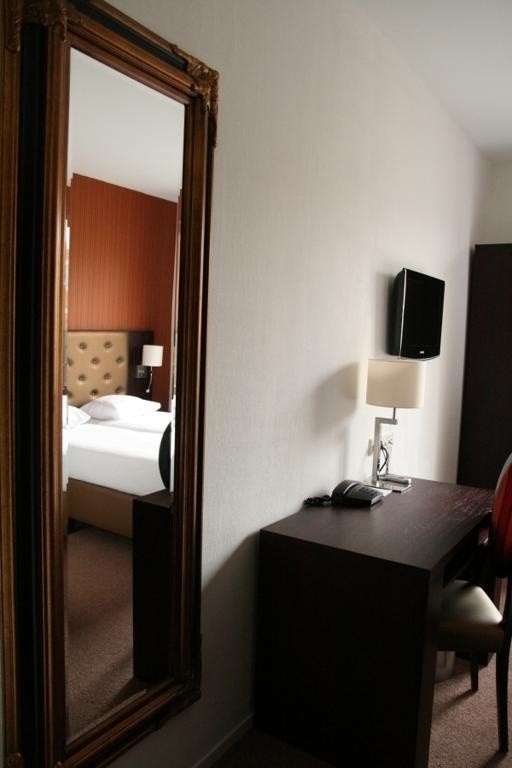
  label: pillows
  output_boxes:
[61,394,161,428]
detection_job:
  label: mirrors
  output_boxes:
[2,0,219,760]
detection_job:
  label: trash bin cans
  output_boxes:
[435,649,455,680]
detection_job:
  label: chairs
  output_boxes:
[437,454,512,757]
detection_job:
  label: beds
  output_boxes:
[62,412,171,539]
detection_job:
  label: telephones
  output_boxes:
[332,480,383,507]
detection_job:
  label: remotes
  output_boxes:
[379,474,411,484]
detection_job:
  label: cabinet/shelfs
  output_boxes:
[132,489,171,685]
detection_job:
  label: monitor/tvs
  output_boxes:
[391,267,446,362]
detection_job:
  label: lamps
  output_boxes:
[367,358,422,491]
[138,343,165,394]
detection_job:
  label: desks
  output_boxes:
[251,478,495,768]
